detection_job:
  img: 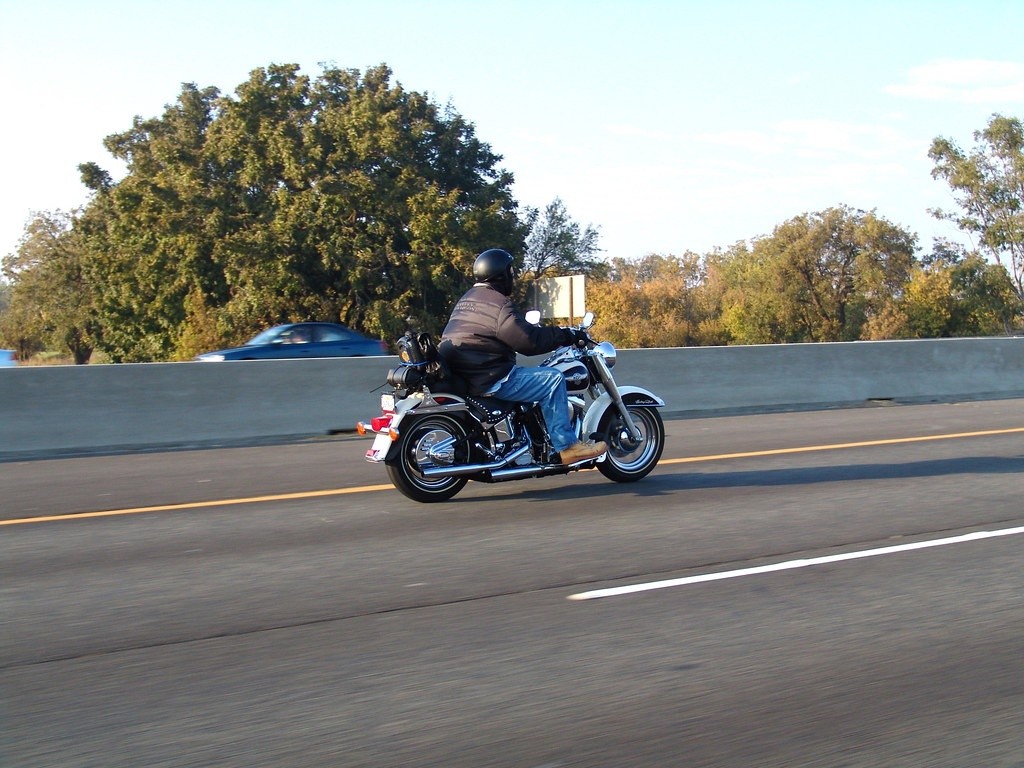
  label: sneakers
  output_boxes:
[560,440,608,465]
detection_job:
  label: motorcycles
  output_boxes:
[356,312,666,503]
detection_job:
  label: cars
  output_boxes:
[194,322,385,362]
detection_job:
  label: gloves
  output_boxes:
[561,327,587,349]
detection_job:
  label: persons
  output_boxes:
[283,335,306,343]
[436,248,610,465]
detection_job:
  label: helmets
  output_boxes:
[473,249,515,283]
